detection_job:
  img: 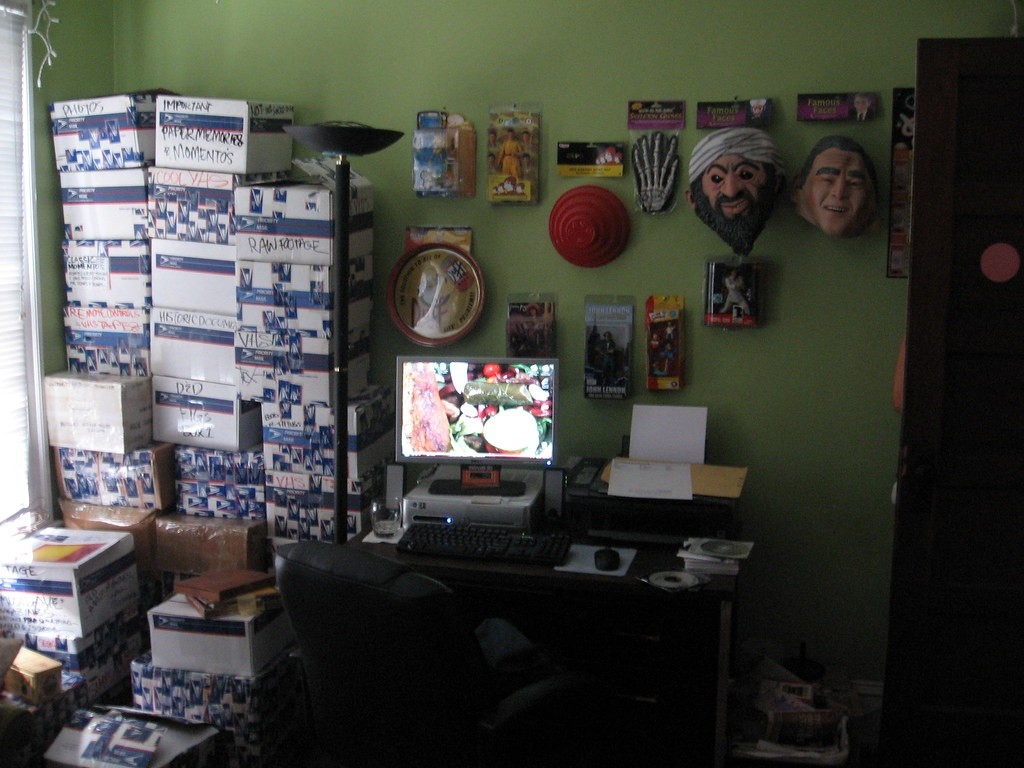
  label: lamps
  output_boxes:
[285,121,403,547]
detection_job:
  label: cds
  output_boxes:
[700,540,748,556]
[650,571,699,588]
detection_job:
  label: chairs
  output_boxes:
[273,540,669,768]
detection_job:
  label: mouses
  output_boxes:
[594,549,621,571]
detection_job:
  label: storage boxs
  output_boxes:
[505,293,556,360]
[584,295,634,398]
[644,295,684,391]
[703,256,763,330]
[0,90,395,768]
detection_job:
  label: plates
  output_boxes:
[385,242,483,345]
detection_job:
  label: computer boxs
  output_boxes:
[402,463,546,529]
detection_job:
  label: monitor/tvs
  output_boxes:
[395,356,560,497]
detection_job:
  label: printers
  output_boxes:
[561,434,745,547]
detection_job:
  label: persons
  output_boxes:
[720,267,750,314]
[849,92,874,121]
[588,325,616,381]
[488,128,533,175]
[528,305,540,317]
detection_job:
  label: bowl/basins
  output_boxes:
[549,185,630,267]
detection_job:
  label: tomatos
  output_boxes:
[483,363,500,377]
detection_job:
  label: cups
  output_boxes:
[370,498,402,538]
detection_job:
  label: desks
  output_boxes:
[345,518,747,768]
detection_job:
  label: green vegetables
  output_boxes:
[433,362,553,458]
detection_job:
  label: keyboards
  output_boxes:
[396,522,571,567]
